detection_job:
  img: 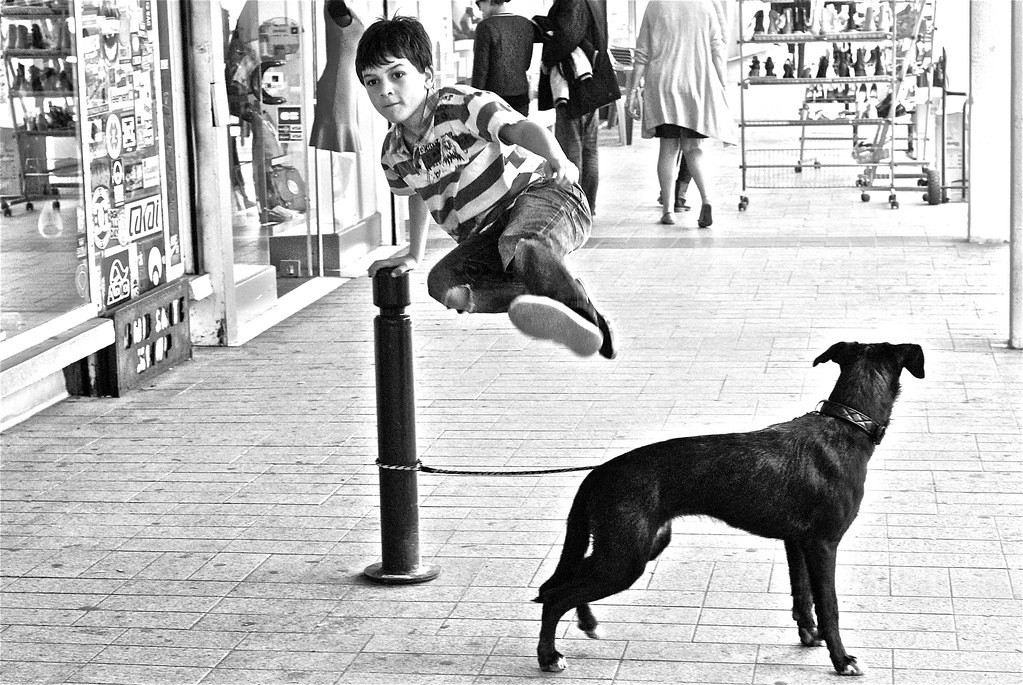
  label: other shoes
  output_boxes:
[1,0,81,140]
[738,1,932,128]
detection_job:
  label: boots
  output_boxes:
[674,179,691,212]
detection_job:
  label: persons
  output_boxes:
[623,1,740,227]
[358,9,618,361]
[307,0,367,224]
[470,1,541,117]
[540,1,622,220]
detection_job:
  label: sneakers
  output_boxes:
[508,276,617,360]
[697,199,713,227]
[660,212,674,224]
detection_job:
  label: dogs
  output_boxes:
[536,341,926,677]
